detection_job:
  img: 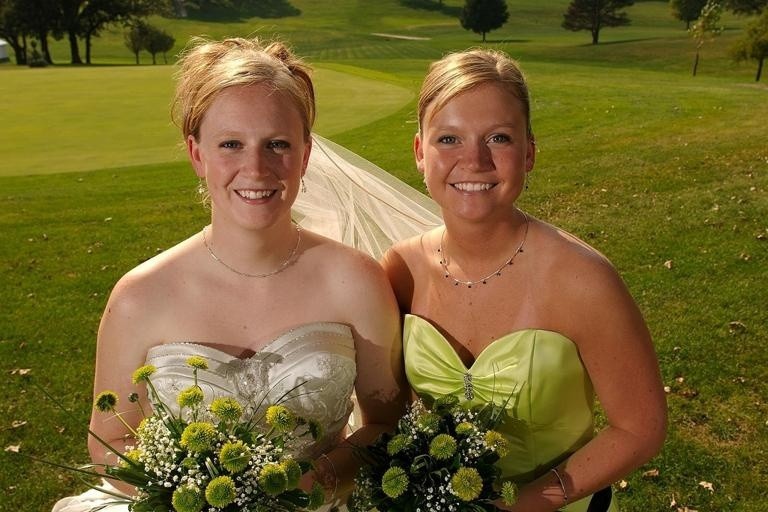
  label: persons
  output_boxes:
[380,47,671,511]
[51,33,444,512]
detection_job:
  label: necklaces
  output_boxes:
[436,205,531,288]
[202,215,301,278]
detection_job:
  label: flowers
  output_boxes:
[350,390,520,511]
[2,359,327,512]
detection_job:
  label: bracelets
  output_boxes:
[319,452,339,505]
[550,466,571,507]
